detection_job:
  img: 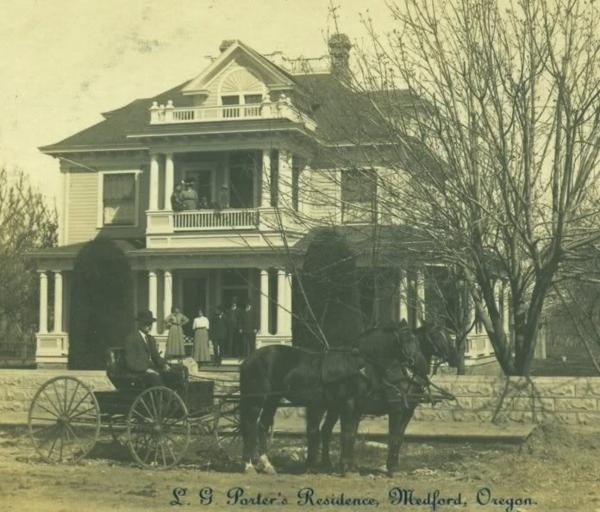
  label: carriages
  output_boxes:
[27,316,461,478]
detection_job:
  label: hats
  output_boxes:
[133,309,156,323]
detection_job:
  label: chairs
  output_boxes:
[105,346,187,392]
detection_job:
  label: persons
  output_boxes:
[168,180,232,212]
[121,309,182,384]
[166,296,256,362]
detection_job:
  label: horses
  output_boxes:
[238,312,458,479]
